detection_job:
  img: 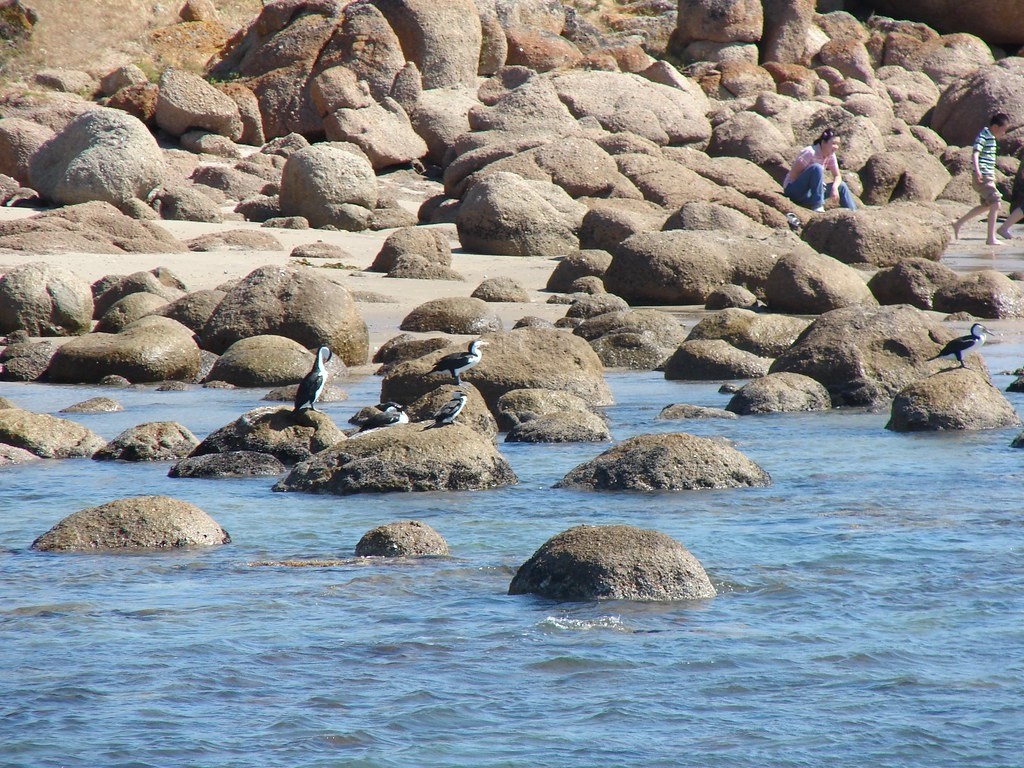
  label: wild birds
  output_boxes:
[358,401,409,432]
[924,323,995,370]
[421,340,490,392]
[420,390,467,430]
[286,344,333,418]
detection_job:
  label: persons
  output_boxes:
[782,128,860,211]
[951,112,1007,247]
[996,140,1024,241]
